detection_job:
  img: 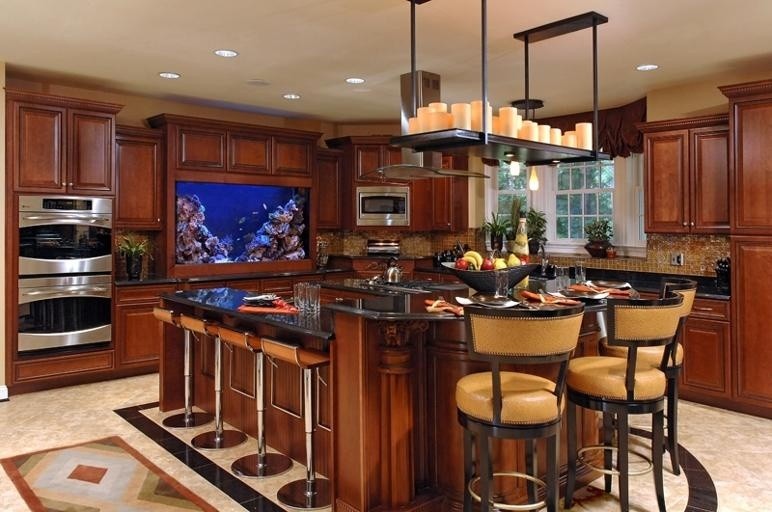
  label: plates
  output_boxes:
[454,296,519,309]
[545,289,610,301]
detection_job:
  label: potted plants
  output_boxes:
[583,216,616,258]
[113,229,161,282]
[478,206,549,259]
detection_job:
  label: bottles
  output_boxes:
[513,217,529,258]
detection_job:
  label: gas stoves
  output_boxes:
[358,274,431,294]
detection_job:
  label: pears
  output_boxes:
[493,257,507,270]
[507,253,522,267]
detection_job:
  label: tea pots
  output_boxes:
[384,256,403,285]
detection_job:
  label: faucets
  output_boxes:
[528,236,549,276]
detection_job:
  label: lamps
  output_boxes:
[389,2,612,167]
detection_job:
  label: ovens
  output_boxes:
[18,194,114,352]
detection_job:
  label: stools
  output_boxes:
[152,306,333,507]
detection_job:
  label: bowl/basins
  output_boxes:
[441,260,542,303]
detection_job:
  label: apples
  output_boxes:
[482,258,493,270]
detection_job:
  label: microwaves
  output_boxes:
[355,186,411,227]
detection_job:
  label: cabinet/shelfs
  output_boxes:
[316,145,347,231]
[324,135,412,187]
[1,86,123,198]
[114,124,165,232]
[148,114,323,188]
[716,76,771,420]
[186,272,352,297]
[632,112,731,236]
[635,294,732,412]
[111,283,176,378]
[407,148,464,234]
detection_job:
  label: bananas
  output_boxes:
[462,250,483,271]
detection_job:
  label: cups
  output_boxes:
[292,281,320,314]
[405,101,594,153]
[555,262,587,290]
[493,263,509,298]
